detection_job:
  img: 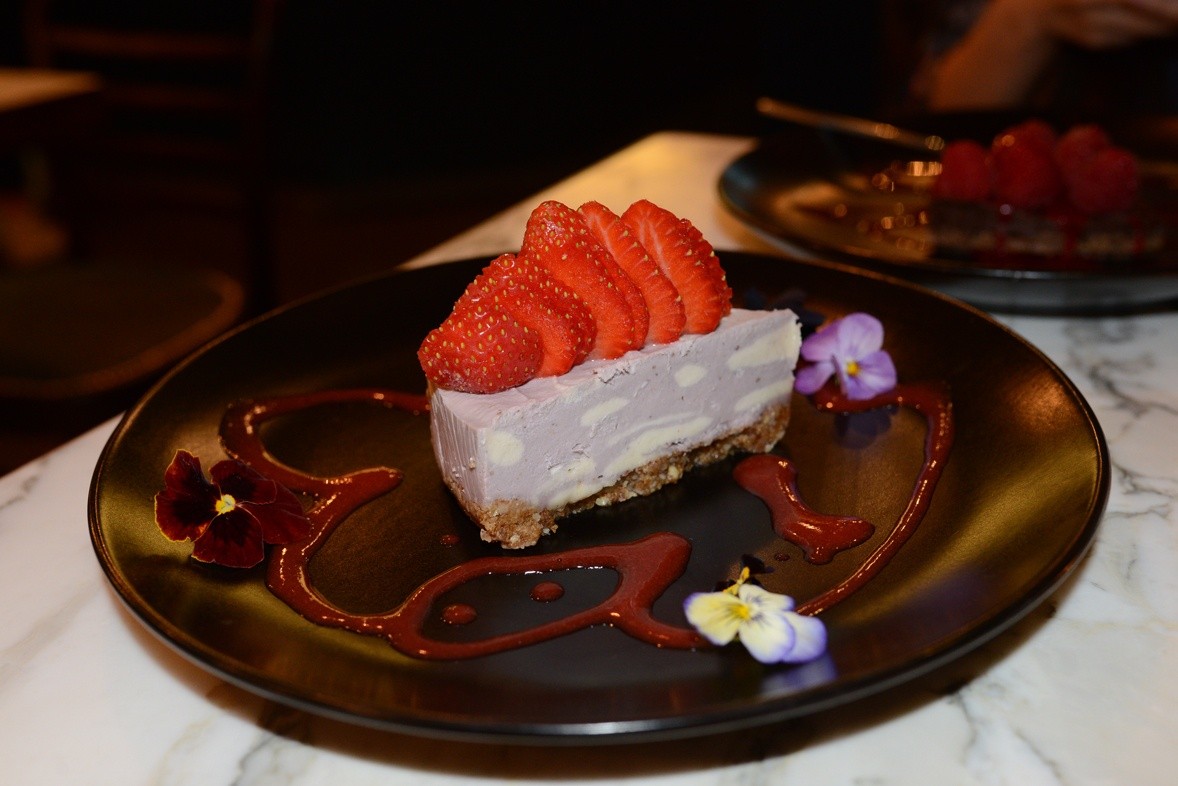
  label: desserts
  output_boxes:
[417,198,804,553]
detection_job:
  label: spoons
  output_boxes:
[754,98,951,155]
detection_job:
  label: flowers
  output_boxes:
[681,566,828,666]
[793,309,901,401]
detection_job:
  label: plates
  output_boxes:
[718,98,1178,320]
[91,238,1110,751]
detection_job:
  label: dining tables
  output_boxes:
[0,126,1178,785]
